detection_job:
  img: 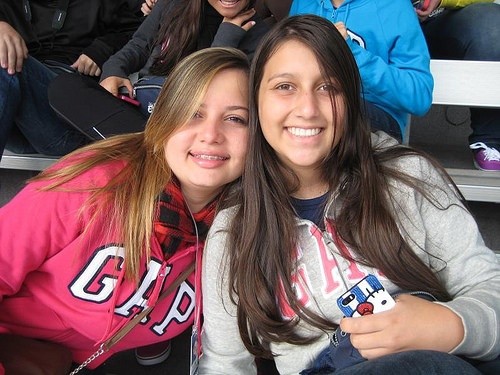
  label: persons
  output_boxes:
[46,0,276,365]
[0,0,147,159]
[139,0,435,144]
[415,0,500,172]
[195,15,500,375]
[0,45,252,375]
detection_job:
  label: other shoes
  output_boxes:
[134,340,172,366]
[446,105,470,125]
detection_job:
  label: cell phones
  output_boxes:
[337,275,396,318]
[117,94,140,106]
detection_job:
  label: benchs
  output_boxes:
[0,59,500,202]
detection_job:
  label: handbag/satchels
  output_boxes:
[132,75,168,118]
[299,291,437,375]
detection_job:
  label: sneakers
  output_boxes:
[469,142,500,171]
